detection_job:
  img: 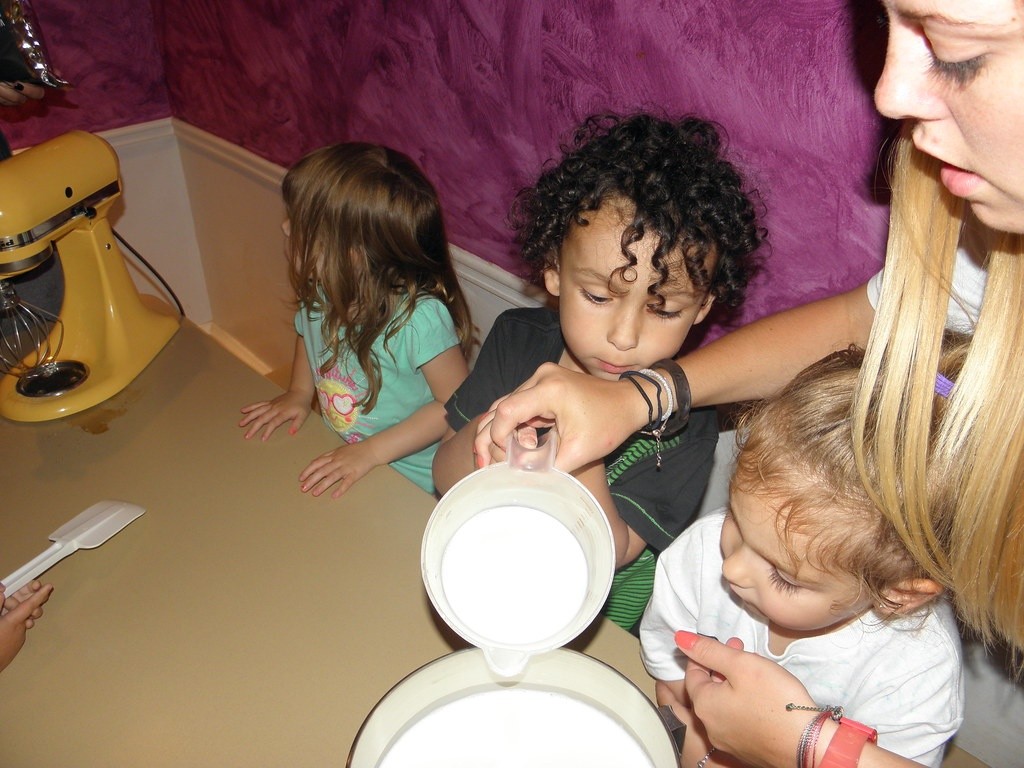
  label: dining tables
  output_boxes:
[2,241,1024,768]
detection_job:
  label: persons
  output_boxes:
[637,336,967,768]
[238,142,475,498]
[0,1,72,161]
[474,1,1024,768]
[0,578,55,674]
[432,106,765,636]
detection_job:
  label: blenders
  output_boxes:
[2,129,181,421]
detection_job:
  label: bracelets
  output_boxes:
[696,747,716,768]
[787,704,878,768]
[618,358,692,473]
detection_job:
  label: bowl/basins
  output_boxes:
[347,647,687,768]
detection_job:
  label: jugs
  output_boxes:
[421,435,616,678]
